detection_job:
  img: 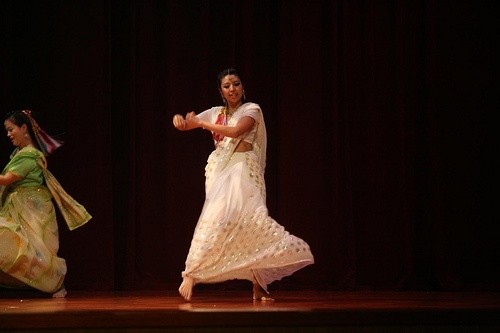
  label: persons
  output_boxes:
[173,68,315,301]
[0,109,92,298]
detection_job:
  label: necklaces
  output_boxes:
[222,103,242,117]
